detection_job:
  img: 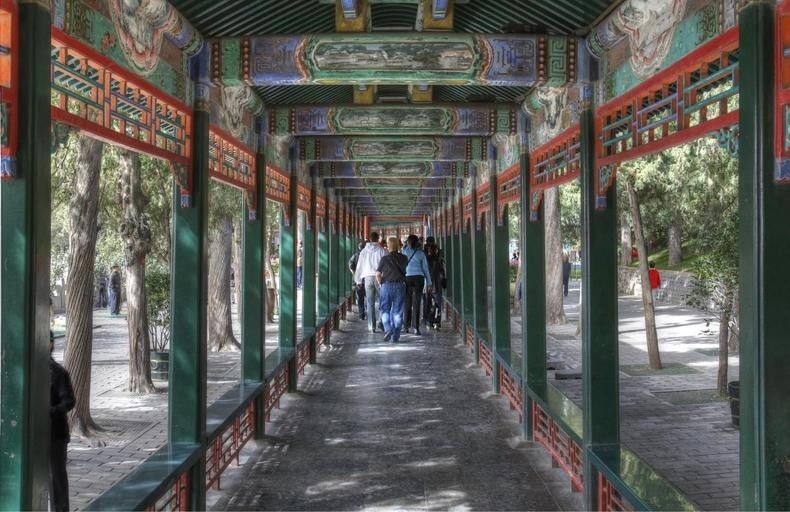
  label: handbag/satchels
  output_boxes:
[422,291,442,323]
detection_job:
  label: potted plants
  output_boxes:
[143,266,169,382]
[685,207,739,429]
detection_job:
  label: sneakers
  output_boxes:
[369,321,441,342]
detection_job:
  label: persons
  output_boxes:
[354,232,390,334]
[105,262,121,313]
[425,245,446,330]
[401,235,431,334]
[421,235,436,327]
[350,241,368,321]
[48,329,77,512]
[563,255,571,295]
[649,261,661,310]
[374,237,408,343]
[295,241,305,287]
